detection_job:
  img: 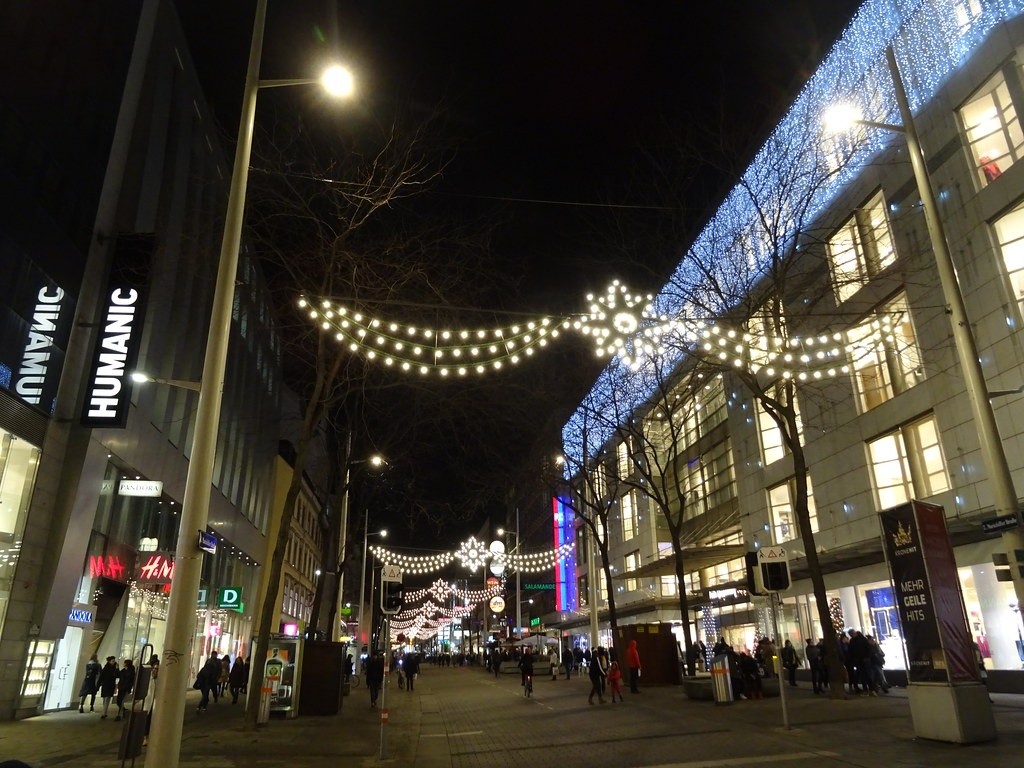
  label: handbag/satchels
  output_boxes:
[194,678,201,690]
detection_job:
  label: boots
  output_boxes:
[588,698,594,705]
[598,696,607,704]
[619,695,624,702]
[855,688,862,694]
[612,695,616,703]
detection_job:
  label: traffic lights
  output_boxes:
[384,581,405,609]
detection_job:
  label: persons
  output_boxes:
[366,649,384,707]
[78,654,160,747]
[425,653,481,667]
[806,630,892,698]
[390,652,421,692]
[345,655,354,682]
[711,638,801,690]
[483,649,538,692]
[196,652,251,713]
[625,641,643,694]
[563,644,624,704]
[693,640,708,671]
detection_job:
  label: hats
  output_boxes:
[90,653,97,658]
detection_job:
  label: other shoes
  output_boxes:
[552,678,556,681]
[214,698,218,703]
[374,701,376,705]
[887,684,892,688]
[792,684,798,686]
[863,689,868,693]
[564,678,570,680]
[372,703,374,707]
[89,707,94,711]
[221,694,223,697]
[818,686,826,693]
[196,706,201,715]
[202,706,207,713]
[217,694,219,696]
[101,715,107,720]
[114,716,121,722]
[122,707,126,718]
[367,685,369,689]
[813,687,819,695]
[79,706,84,713]
[881,686,889,694]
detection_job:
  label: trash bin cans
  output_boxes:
[257,678,273,724]
[116,710,148,761]
[709,652,734,703]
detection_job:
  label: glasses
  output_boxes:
[379,653,383,656]
[721,640,724,642]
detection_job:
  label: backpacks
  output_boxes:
[398,674,405,689]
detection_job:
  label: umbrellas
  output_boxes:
[501,633,558,645]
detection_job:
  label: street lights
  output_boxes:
[130,12,356,768]
[818,41,1024,621]
[331,424,384,640]
[358,509,388,644]
[496,508,535,640]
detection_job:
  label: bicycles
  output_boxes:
[395,668,405,689]
[524,675,533,697]
[346,672,360,689]
